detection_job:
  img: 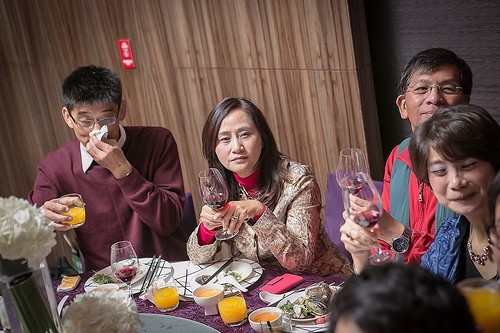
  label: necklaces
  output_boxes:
[467,234,493,267]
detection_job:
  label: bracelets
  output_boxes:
[125,171,131,177]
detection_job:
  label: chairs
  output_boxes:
[177,190,197,237]
[324,173,385,257]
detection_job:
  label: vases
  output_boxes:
[0,257,65,333]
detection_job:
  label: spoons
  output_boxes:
[218,276,248,293]
[259,288,295,304]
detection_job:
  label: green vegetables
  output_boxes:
[91,274,114,284]
[280,299,314,317]
[225,272,242,287]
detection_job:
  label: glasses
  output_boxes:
[66,102,120,130]
[405,81,465,95]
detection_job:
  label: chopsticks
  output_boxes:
[202,250,242,285]
[139,254,162,295]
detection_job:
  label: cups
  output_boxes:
[217,290,248,327]
[457,277,500,333]
[58,193,85,227]
[151,276,180,312]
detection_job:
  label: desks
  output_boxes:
[0,257,350,333]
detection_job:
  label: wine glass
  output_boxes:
[339,172,396,267]
[334,147,368,227]
[110,241,146,312]
[197,168,239,242]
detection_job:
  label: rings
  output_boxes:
[348,228,353,237]
[231,216,238,222]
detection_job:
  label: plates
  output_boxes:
[173,257,263,297]
[84,257,173,295]
[278,285,340,322]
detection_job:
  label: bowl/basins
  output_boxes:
[248,307,282,333]
[192,284,225,308]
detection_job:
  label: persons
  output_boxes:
[186,97,354,275]
[407,103,500,285]
[27,65,189,271]
[339,48,473,276]
[330,263,479,333]
[489,172,500,244]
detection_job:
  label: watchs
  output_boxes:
[392,225,412,253]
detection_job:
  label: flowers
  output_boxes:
[0,195,57,271]
